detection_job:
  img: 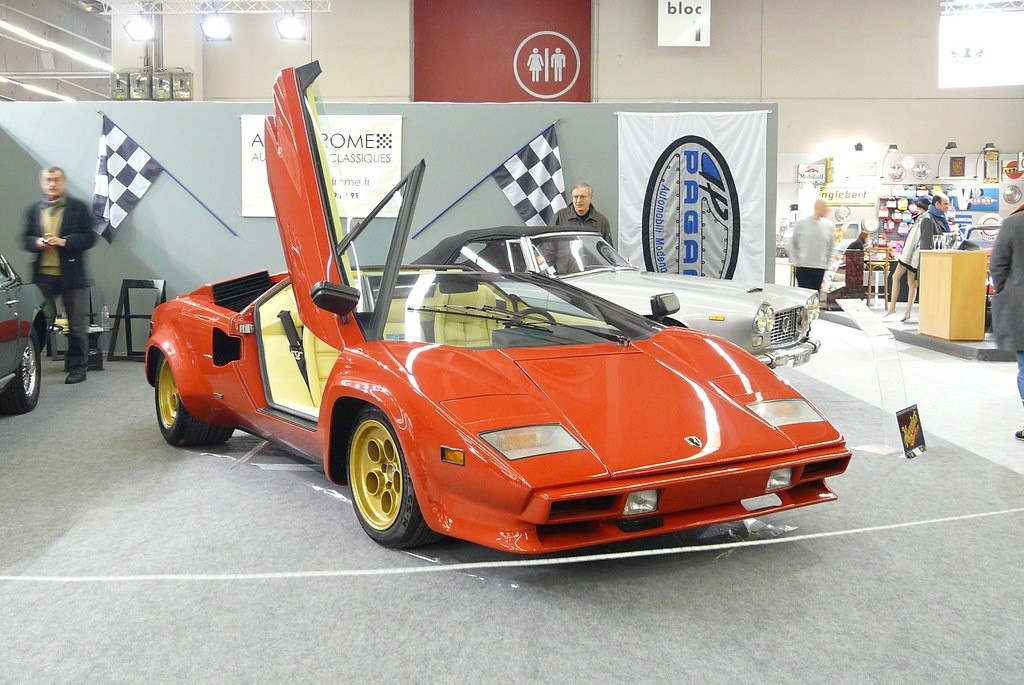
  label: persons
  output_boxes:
[989,202,1024,441]
[543,183,616,275]
[847,231,870,249]
[22,166,95,384]
[788,199,835,295]
[921,191,952,250]
[883,193,935,322]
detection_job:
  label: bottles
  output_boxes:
[102,302,109,326]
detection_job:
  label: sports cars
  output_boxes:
[404,225,824,371]
[145,57,853,556]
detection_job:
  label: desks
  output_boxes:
[53,325,117,372]
[789,247,890,311]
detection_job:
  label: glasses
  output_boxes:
[571,193,591,200]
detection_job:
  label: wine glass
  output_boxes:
[933,235,942,250]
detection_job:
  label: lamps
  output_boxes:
[277,11,307,41]
[972,141,997,178]
[935,141,959,179]
[879,145,898,178]
[122,11,158,42]
[200,6,232,42]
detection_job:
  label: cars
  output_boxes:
[0,256,64,416]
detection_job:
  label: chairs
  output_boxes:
[303,322,341,408]
[434,287,499,347]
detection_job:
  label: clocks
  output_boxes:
[861,215,880,233]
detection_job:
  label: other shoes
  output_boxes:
[65,371,86,383]
[1015,430,1024,441]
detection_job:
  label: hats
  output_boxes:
[914,195,933,211]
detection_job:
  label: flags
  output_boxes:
[491,125,568,226]
[91,116,163,244]
